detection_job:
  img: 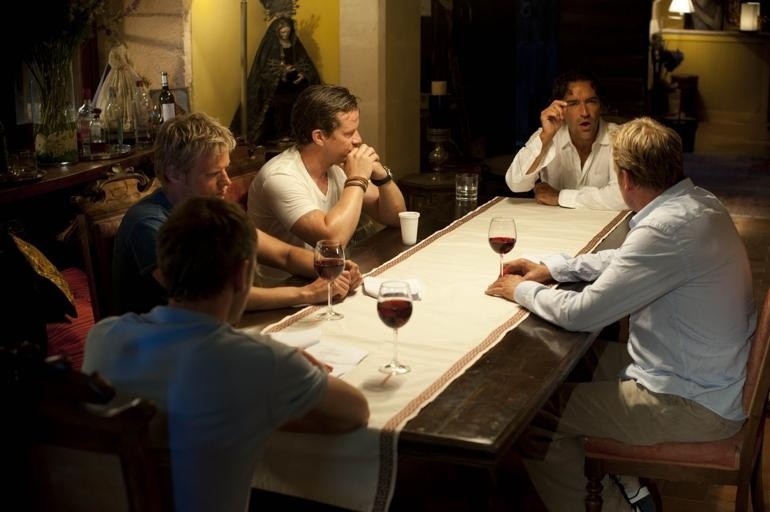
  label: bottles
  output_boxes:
[78,69,174,162]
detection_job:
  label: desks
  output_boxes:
[0,146,154,196]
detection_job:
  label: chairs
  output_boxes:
[580,296,770,509]
[71,178,170,327]
[8,372,174,512]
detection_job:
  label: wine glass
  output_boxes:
[314,238,345,320]
[374,281,416,374]
[488,215,519,278]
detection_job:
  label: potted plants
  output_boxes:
[7,3,139,165]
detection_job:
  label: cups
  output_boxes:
[452,172,481,207]
[399,210,420,246]
[11,150,40,180]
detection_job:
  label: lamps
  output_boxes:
[666,0,702,19]
[738,3,763,36]
[417,64,461,173]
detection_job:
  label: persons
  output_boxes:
[505,64,632,211]
[82,198,370,511]
[99,112,364,315]
[488,117,757,511]
[229,15,327,148]
[247,83,410,286]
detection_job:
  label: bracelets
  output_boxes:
[369,166,393,186]
[344,176,369,193]
